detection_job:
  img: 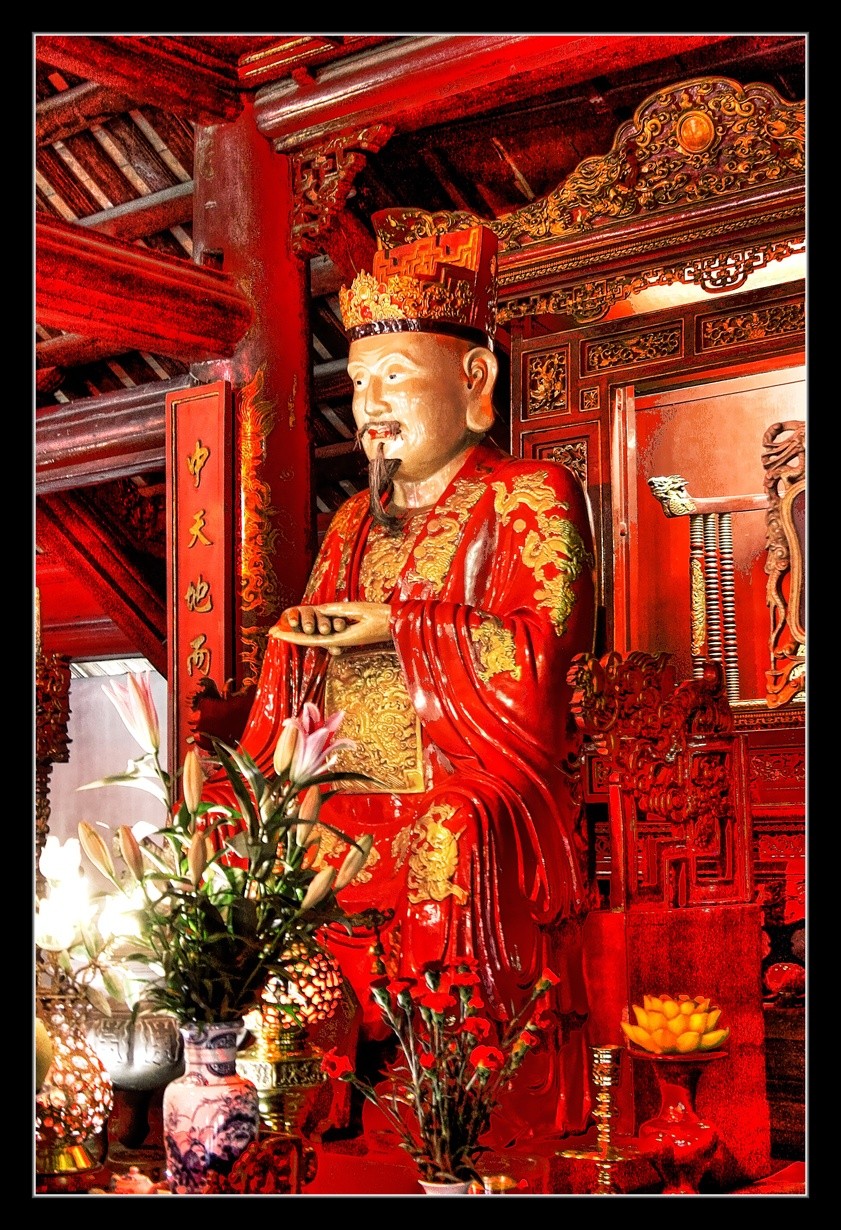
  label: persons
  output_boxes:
[193,227,595,1194]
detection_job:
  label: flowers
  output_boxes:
[31,677,582,1185]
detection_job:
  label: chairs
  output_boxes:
[185,652,773,1184]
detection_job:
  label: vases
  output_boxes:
[631,1040,728,1196]
[30,985,473,1195]
